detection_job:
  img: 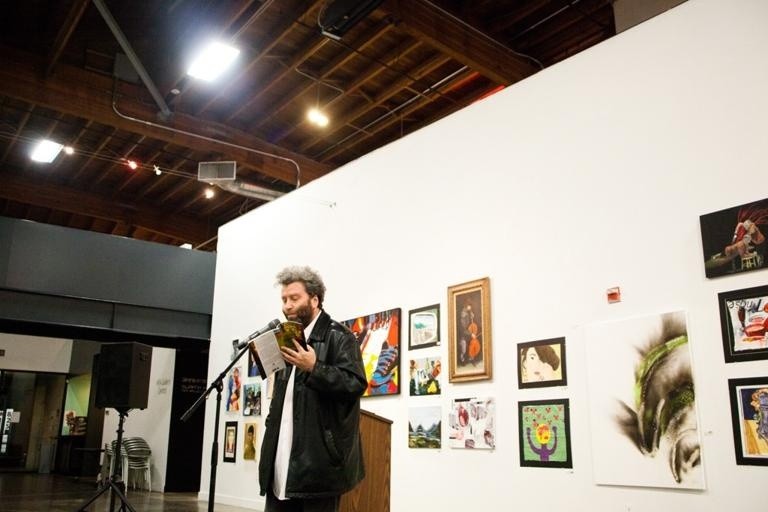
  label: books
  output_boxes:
[247,321,309,381]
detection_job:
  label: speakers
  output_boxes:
[95,341,152,409]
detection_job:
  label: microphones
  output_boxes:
[238,319,280,349]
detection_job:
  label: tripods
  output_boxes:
[79,409,137,512]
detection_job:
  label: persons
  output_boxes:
[258,265,369,512]
[458,298,477,368]
[522,345,560,382]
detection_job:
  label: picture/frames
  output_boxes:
[447,276,492,383]
[408,304,440,350]
[518,399,572,469]
[719,285,768,363]
[517,337,567,389]
[223,421,238,462]
[728,376,768,465]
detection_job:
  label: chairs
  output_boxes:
[104,437,152,496]
